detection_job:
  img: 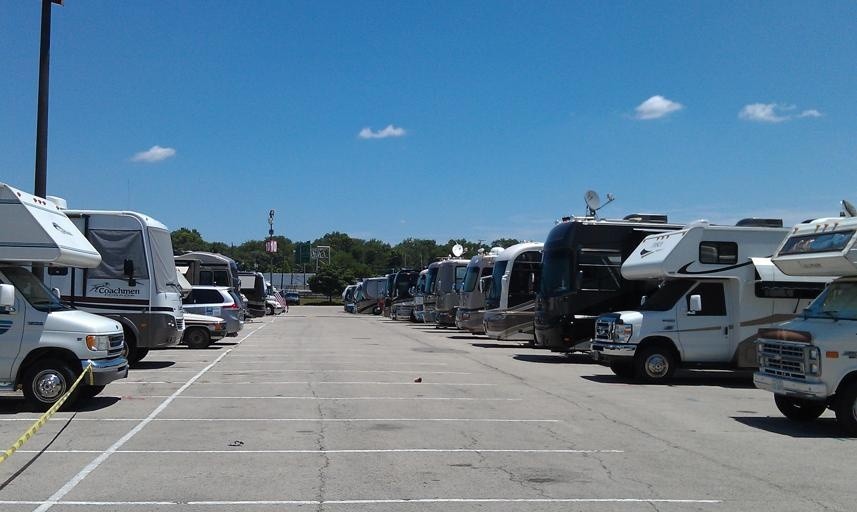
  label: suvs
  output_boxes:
[182,283,245,348]
[284,291,300,305]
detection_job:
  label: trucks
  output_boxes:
[238,270,286,320]
[751,199,856,432]
[590,219,841,383]
[0,182,131,411]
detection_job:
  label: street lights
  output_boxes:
[268,209,274,286]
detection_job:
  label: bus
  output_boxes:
[19,195,185,365]
[341,259,470,325]
[533,190,784,358]
[479,240,545,344]
[454,247,498,335]
[173,248,242,306]
